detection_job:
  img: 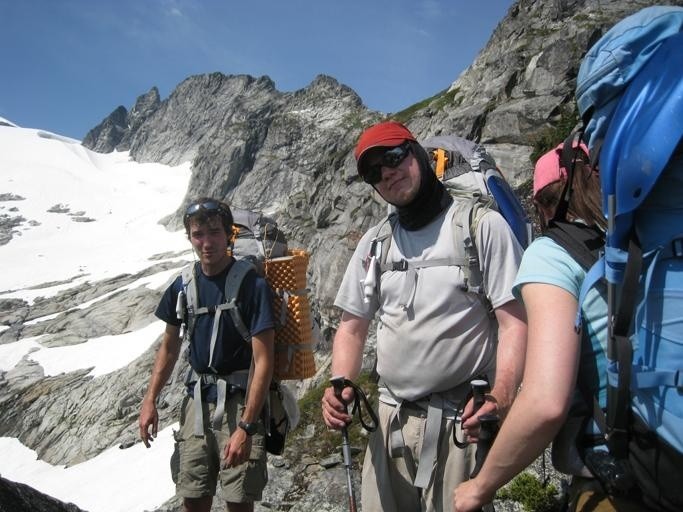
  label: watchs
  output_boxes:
[238,419,258,436]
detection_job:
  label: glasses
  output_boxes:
[183,200,228,224]
[358,141,414,185]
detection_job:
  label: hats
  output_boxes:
[354,120,418,180]
[532,139,594,196]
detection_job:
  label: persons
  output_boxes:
[319,121,525,512]
[450,138,620,512]
[137,198,279,512]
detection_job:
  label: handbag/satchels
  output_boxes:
[230,205,288,260]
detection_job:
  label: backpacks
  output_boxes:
[564,9,681,511]
[361,129,534,490]
